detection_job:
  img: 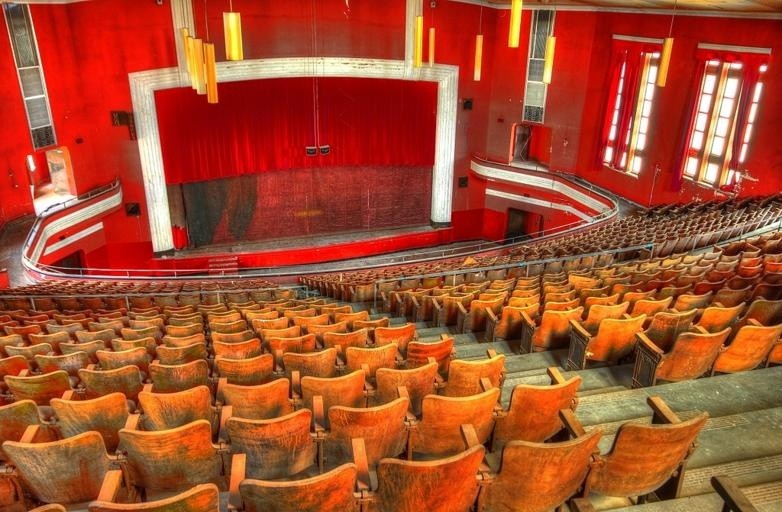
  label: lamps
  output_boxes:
[416,1,425,67]
[542,0,557,84]
[429,1,436,67]
[474,1,483,81]
[509,0,523,49]
[191,2,206,95]
[186,1,197,90]
[198,0,220,103]
[181,2,191,72]
[656,2,679,87]
[223,1,245,61]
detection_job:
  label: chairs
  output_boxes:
[99,317,129,324]
[61,318,95,327]
[111,338,158,357]
[508,294,542,307]
[127,310,159,319]
[519,306,584,354]
[701,285,753,307]
[0,464,67,512]
[3,325,45,342]
[284,309,318,325]
[631,324,733,387]
[0,320,19,331]
[610,296,676,318]
[89,313,122,322]
[118,405,232,490]
[581,286,619,305]
[432,294,478,327]
[264,301,296,311]
[309,321,346,339]
[207,309,237,315]
[351,424,492,512]
[320,329,370,363]
[298,299,324,309]
[636,284,693,308]
[276,349,338,378]
[0,310,26,320]
[559,397,708,500]
[335,311,371,330]
[479,367,581,443]
[619,308,698,360]
[565,301,635,348]
[146,359,214,403]
[121,326,161,340]
[412,293,450,320]
[29,331,75,353]
[299,193,782,299]
[247,317,289,334]
[59,340,105,360]
[240,309,270,317]
[668,302,746,334]
[88,320,125,336]
[293,370,367,429]
[544,290,579,300]
[138,382,218,436]
[196,304,225,308]
[0,334,28,357]
[4,369,79,405]
[210,338,268,360]
[45,323,88,340]
[164,305,194,309]
[170,313,201,316]
[0,355,33,391]
[334,343,399,374]
[257,326,305,345]
[50,390,136,452]
[88,452,246,512]
[0,399,54,459]
[246,311,278,325]
[562,312,647,371]
[1,280,290,303]
[398,386,501,460]
[239,463,358,512]
[198,307,226,317]
[276,304,307,316]
[227,301,256,310]
[310,302,337,315]
[456,297,505,332]
[23,320,57,333]
[321,305,352,323]
[95,347,151,377]
[162,335,207,347]
[2,425,118,504]
[766,337,782,368]
[485,304,540,342]
[264,332,317,367]
[615,288,657,314]
[131,306,160,312]
[208,313,241,323]
[353,316,389,335]
[29,309,60,319]
[78,364,146,400]
[745,283,782,301]
[726,295,782,344]
[394,291,426,315]
[64,310,93,318]
[373,322,420,360]
[213,355,276,385]
[396,333,457,371]
[712,317,782,379]
[166,323,205,337]
[523,298,581,325]
[289,313,334,335]
[258,298,285,310]
[480,444,603,512]
[74,328,119,347]
[34,351,91,376]
[0,315,12,321]
[4,343,53,365]
[156,343,208,361]
[134,314,166,320]
[164,308,193,315]
[224,396,318,479]
[168,316,204,327]
[55,313,86,325]
[380,289,413,313]
[129,318,167,333]
[562,293,620,320]
[207,330,254,342]
[649,290,713,311]
[313,395,410,464]
[364,361,438,416]
[205,320,247,337]
[688,286,751,307]
[16,315,49,325]
[215,379,293,427]
[96,307,127,316]
[428,349,508,411]
[231,304,261,313]
